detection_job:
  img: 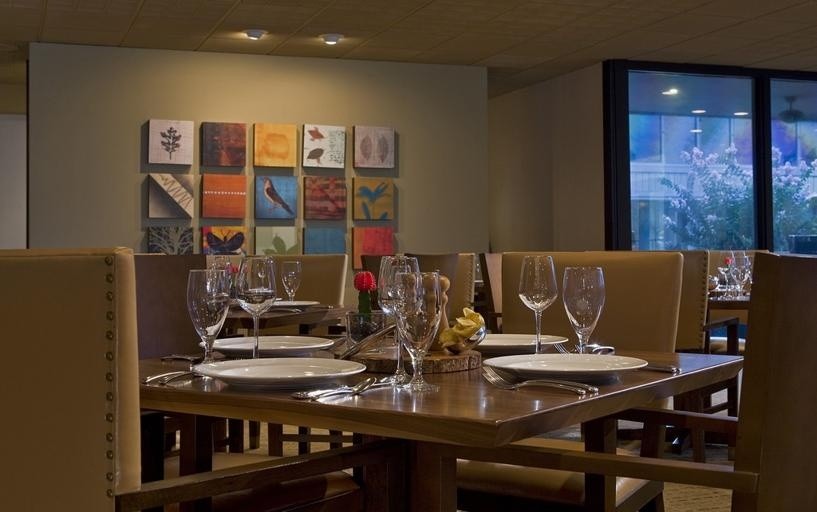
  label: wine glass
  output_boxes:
[280,260,302,300]
[378,254,441,394]
[559,265,608,357]
[708,250,753,299]
[517,255,557,355]
[185,268,231,363]
[232,254,277,356]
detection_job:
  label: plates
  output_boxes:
[270,300,319,307]
[479,332,567,352]
[201,334,334,357]
[481,353,647,381]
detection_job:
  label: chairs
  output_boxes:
[0,245,817,511]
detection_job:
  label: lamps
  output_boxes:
[320,33,345,45]
[243,28,269,40]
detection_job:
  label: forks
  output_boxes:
[194,355,366,389]
[162,351,201,365]
[553,343,570,353]
[482,367,598,398]
[142,363,204,388]
[290,377,395,399]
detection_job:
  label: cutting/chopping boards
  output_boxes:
[336,348,481,373]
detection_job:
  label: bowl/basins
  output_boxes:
[445,324,486,355]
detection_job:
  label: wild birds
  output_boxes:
[259,177,294,215]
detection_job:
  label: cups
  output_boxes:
[204,255,274,312]
[344,310,389,355]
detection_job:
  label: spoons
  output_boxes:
[308,376,374,402]
